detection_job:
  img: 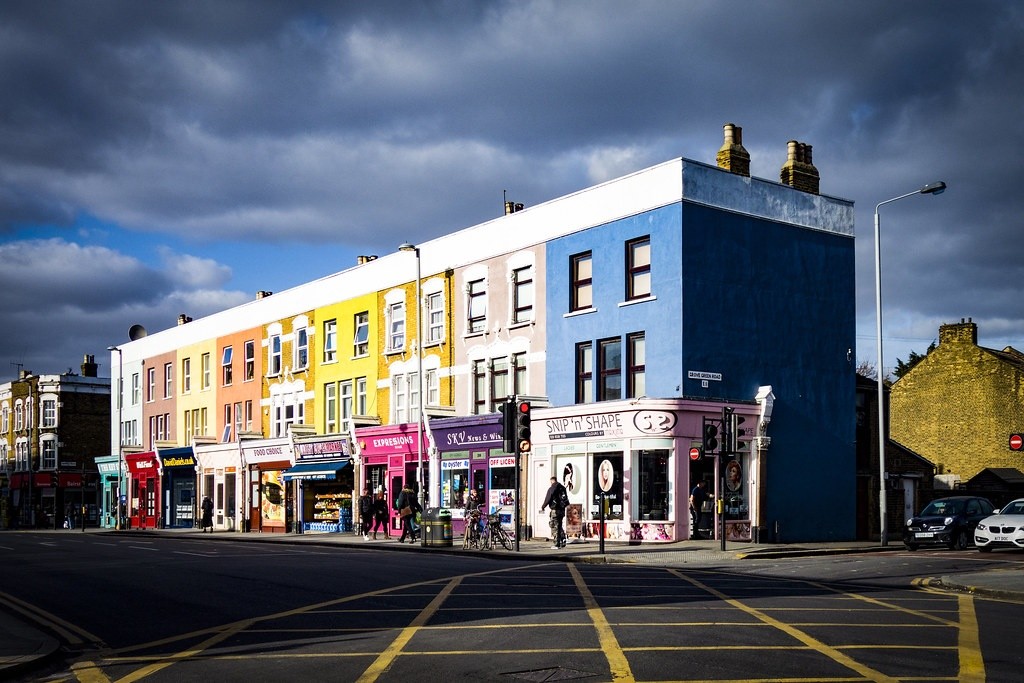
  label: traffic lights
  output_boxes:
[51,469,59,488]
[516,401,531,453]
[735,416,745,452]
[706,424,718,452]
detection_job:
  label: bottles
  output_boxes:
[310,522,337,532]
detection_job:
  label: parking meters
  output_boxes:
[81,505,85,532]
[717,495,725,550]
[600,492,609,554]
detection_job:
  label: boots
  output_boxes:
[373,532,376,540]
[210,528,213,533]
[202,529,206,532]
[384,532,391,539]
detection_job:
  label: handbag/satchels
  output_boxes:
[401,505,412,518]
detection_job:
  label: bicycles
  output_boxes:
[464,503,513,550]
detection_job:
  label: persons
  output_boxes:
[501,492,514,505]
[398,484,423,543]
[609,524,673,540]
[727,524,750,539]
[202,496,214,532]
[601,462,610,490]
[729,462,741,489]
[564,463,573,491]
[358,489,391,540]
[689,482,714,538]
[66,502,77,529]
[568,506,580,537]
[463,490,479,544]
[542,477,570,548]
[588,523,599,538]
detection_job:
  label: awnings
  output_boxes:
[282,460,350,481]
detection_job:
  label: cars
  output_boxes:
[974,498,1024,553]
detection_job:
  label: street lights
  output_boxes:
[19,379,32,524]
[875,181,948,547]
[107,344,123,530]
[398,241,425,511]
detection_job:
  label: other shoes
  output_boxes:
[562,539,566,547]
[551,545,558,550]
[363,531,370,541]
[398,538,404,543]
[409,541,414,544]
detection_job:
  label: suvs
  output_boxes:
[904,496,996,551]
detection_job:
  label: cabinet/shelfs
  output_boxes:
[314,495,351,520]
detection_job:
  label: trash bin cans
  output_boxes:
[420,507,453,548]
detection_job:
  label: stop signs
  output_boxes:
[690,448,699,461]
[1010,435,1022,450]
